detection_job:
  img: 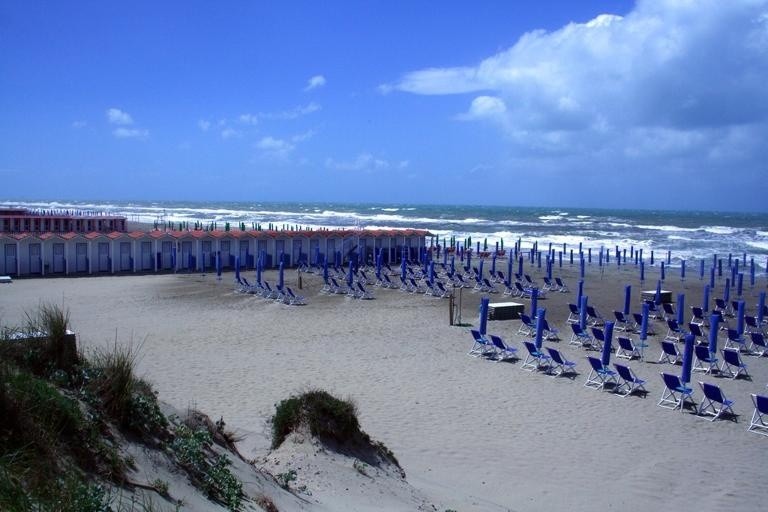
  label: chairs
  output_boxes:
[235,262,569,307]
[546,345,578,378]
[611,364,647,398]
[534,316,560,341]
[657,371,696,412]
[697,381,738,424]
[691,343,720,377]
[720,346,752,380]
[584,356,616,391]
[746,392,767,435]
[517,311,536,337]
[466,328,493,356]
[566,290,767,364]
[520,339,552,372]
[487,333,518,364]
